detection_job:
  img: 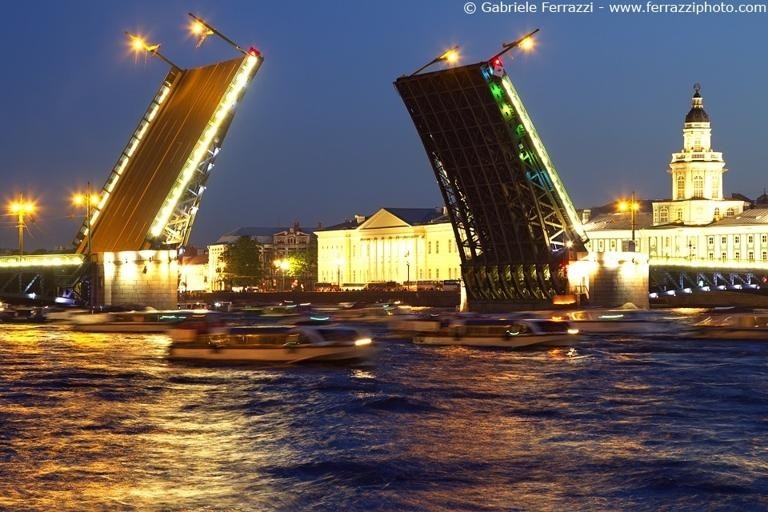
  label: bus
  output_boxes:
[232,275,259,293]
[313,280,460,292]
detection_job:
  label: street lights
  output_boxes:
[335,258,343,287]
[71,181,100,253]
[497,28,540,57]
[615,191,641,241]
[7,193,38,255]
[189,12,248,55]
[406,260,411,290]
[412,46,459,74]
[125,31,184,74]
[274,260,290,289]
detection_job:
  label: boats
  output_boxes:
[165,304,389,372]
[566,310,684,334]
[276,303,441,339]
[0,303,52,323]
[401,312,573,352]
[75,301,229,333]
